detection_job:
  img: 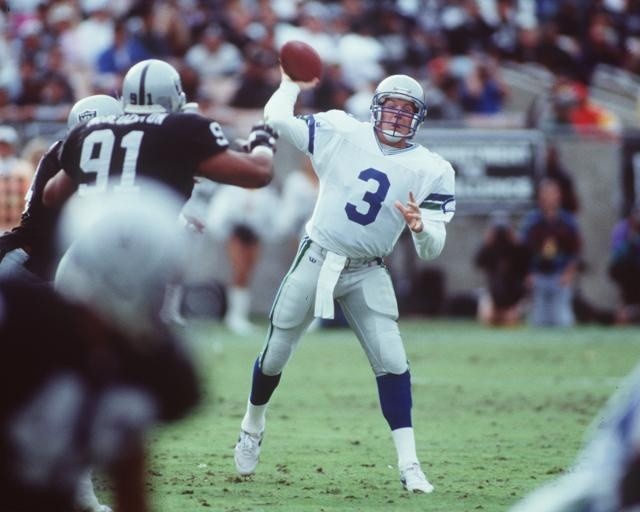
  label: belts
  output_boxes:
[306,241,379,271]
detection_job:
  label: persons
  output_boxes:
[609,207,640,325]
[0,60,278,512]
[474,148,586,329]
[277,154,319,245]
[0,0,640,130]
[233,56,456,494]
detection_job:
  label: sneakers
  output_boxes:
[72,476,112,512]
[398,462,433,492]
[234,419,265,477]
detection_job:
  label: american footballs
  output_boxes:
[281,41,321,80]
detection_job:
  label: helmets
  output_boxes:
[123,60,184,117]
[68,93,123,135]
[369,74,428,145]
[53,179,198,342]
